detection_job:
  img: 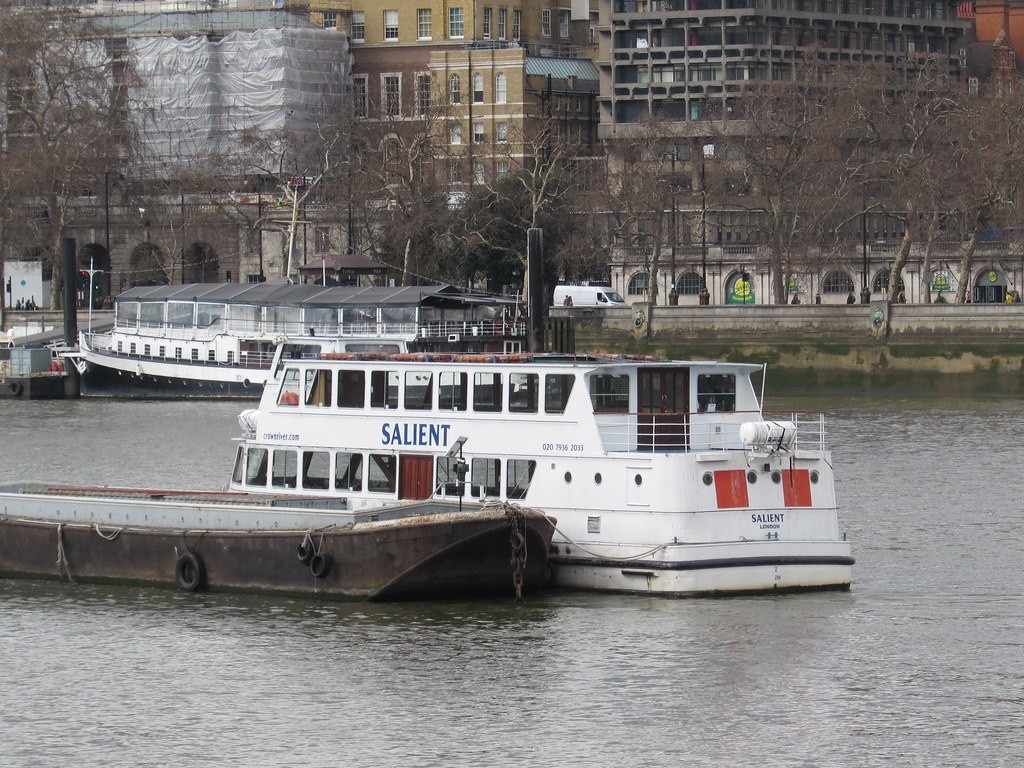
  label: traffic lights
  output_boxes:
[81,272,87,288]
[95,282,99,290]
[7,284,10,292]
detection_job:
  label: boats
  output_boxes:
[0,436,558,602]
[228,353,856,598]
[79,283,529,399]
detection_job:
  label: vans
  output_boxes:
[553,285,626,306]
